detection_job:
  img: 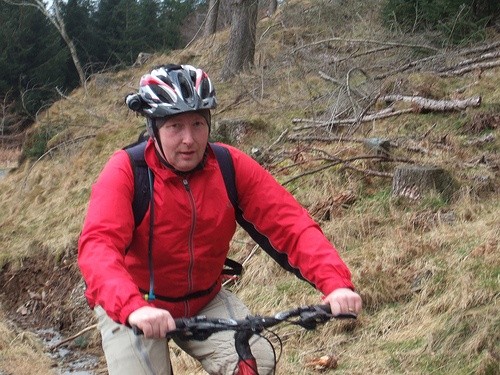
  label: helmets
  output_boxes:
[124,62,219,119]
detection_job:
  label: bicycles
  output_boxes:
[133,302,358,375]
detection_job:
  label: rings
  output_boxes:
[348,307,355,312]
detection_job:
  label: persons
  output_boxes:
[77,63,363,375]
